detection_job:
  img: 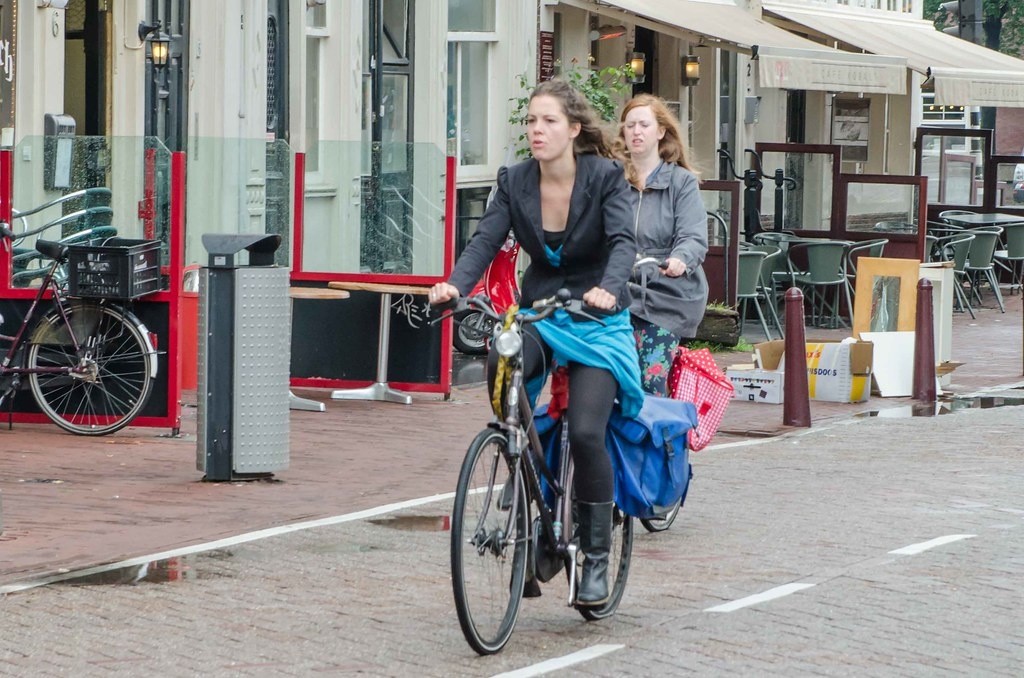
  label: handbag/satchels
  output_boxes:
[529,395,698,521]
[669,344,733,453]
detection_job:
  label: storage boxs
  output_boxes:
[752,337,874,403]
[725,369,785,404]
[67,236,162,299]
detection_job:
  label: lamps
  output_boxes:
[138,18,171,73]
[681,54,700,86]
[827,90,840,97]
[626,51,646,84]
[590,22,627,40]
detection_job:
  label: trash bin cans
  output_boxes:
[194,232,291,485]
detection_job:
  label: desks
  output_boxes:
[328,281,431,404]
[938,212,1024,288]
[289,286,352,411]
[756,235,856,246]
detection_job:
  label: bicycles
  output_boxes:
[424,287,633,655]
[614,252,694,534]
[0,219,163,437]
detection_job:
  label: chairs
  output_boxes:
[738,209,1024,342]
[9,187,118,287]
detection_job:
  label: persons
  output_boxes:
[427,79,638,605]
[609,93,710,399]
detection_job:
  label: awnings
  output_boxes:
[559,0,909,95]
[743,0,1024,108]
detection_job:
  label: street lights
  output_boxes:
[147,17,171,263]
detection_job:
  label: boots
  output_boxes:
[574,498,614,607]
[497,471,522,510]
